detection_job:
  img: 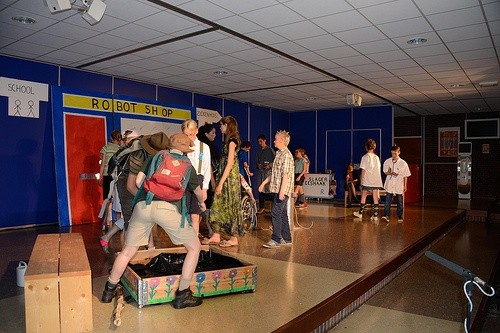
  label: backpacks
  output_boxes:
[107,135,145,178]
[143,152,190,201]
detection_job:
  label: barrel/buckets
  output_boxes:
[16,261,27,287]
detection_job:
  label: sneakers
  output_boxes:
[173,286,203,309]
[353,211,362,218]
[102,281,117,303]
[262,239,280,248]
[370,215,378,221]
[280,238,291,245]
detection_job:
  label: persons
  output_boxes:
[256,135,275,214]
[102,133,207,309]
[99,120,256,256]
[258,130,294,248]
[294,149,308,208]
[345,163,361,208]
[353,139,383,220]
[301,149,310,174]
[201,117,242,247]
[382,146,411,224]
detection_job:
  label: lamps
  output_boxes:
[80,0,107,26]
[46,0,72,14]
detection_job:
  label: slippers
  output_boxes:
[219,240,239,247]
[202,239,220,244]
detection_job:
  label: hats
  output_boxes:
[141,131,170,155]
[165,133,195,153]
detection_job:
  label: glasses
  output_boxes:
[219,124,224,126]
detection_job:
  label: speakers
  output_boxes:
[82,0,107,25]
[45,0,71,13]
[345,94,362,107]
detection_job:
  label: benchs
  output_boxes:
[345,188,405,219]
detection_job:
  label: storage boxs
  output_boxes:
[114,244,258,308]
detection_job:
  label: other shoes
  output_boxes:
[398,217,403,222]
[144,246,156,251]
[257,208,265,214]
[299,204,308,209]
[381,216,389,223]
[99,236,110,255]
[265,209,271,212]
[105,225,110,230]
